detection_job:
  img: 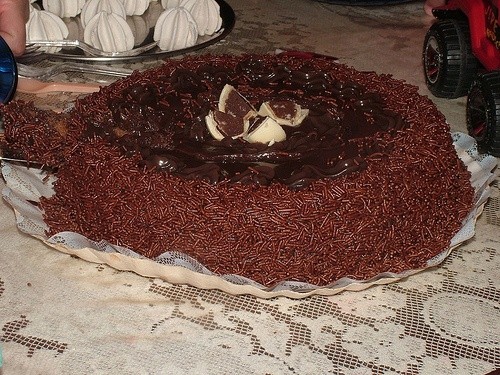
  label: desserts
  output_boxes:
[25,0,223,53]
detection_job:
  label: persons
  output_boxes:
[0,0,31,58]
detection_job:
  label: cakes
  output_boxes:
[0,50,477,286]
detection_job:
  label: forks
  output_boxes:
[15,61,134,81]
[24,37,159,56]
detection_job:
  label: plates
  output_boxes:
[25,1,239,60]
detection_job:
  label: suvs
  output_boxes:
[422,0,500,159]
